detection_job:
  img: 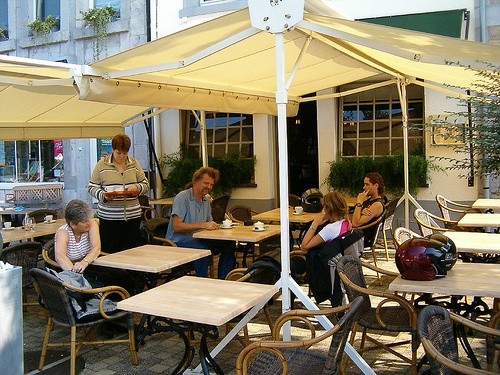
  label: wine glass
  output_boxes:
[26,217,36,233]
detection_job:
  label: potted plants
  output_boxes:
[25,14,60,54]
[79,5,117,60]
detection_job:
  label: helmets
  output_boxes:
[424,234,457,271]
[302,188,324,213]
[395,237,447,281]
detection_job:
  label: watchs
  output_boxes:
[356,202,363,208]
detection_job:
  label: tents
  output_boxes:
[0,0,500,375]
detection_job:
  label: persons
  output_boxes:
[351,171,385,252]
[290,191,353,277]
[162,166,237,280]
[87,133,151,255]
[52,198,133,339]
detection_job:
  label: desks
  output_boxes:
[37,216,99,229]
[148,196,175,215]
[192,223,280,269]
[471,198,500,214]
[90,243,212,341]
[457,213,500,233]
[442,231,500,261]
[0,226,60,246]
[388,262,500,345]
[116,275,280,375]
[252,204,327,252]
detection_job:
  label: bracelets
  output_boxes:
[83,258,91,265]
[308,225,318,232]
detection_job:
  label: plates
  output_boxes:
[252,228,265,231]
[44,220,56,223]
[2,227,15,230]
[292,213,305,215]
[222,227,232,229]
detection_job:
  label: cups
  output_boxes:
[223,218,233,226]
[4,222,12,228]
[295,206,303,213]
[105,187,115,192]
[253,221,264,229]
[44,215,53,222]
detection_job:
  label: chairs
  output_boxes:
[0,192,500,375]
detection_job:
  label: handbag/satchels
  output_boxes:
[57,269,120,319]
